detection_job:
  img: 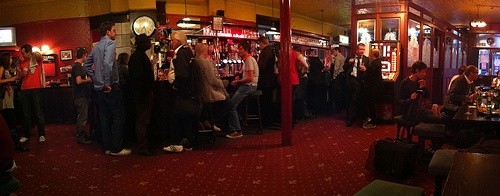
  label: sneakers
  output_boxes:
[104,147,132,156]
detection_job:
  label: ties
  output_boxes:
[356,56,361,76]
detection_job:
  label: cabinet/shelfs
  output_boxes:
[186,34,258,72]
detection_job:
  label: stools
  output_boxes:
[414,122,446,167]
[242,89,263,134]
[429,149,457,196]
[196,100,216,146]
[354,179,423,196]
[394,115,414,141]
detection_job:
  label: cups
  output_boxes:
[416,90,422,105]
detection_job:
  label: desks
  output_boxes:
[12,86,76,123]
[451,105,500,140]
[442,151,500,196]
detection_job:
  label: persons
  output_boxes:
[0,44,47,196]
[221,41,259,138]
[71,22,229,156]
[491,70,500,90]
[396,61,432,120]
[256,37,282,128]
[445,64,480,110]
[291,43,383,129]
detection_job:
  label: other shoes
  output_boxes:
[137,148,157,156]
[19,137,29,143]
[15,145,28,152]
[38,135,46,143]
[346,118,352,127]
[362,118,377,129]
[197,120,244,139]
[159,139,193,153]
[77,134,93,144]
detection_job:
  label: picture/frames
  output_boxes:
[60,49,73,61]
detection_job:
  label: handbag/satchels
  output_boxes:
[0,67,9,100]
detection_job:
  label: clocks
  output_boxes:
[132,15,156,39]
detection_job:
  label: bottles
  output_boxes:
[187,37,260,77]
[476,88,499,119]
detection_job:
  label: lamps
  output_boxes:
[471,4,487,28]
